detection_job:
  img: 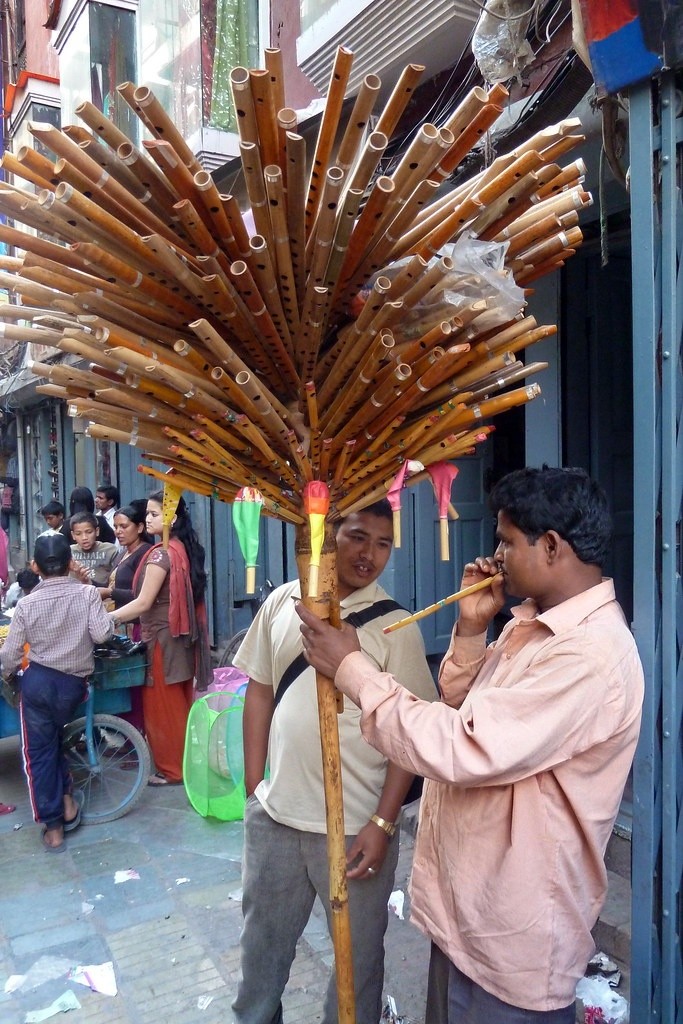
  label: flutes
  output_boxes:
[381,571,503,634]
[0,44,594,601]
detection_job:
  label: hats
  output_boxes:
[34,531,71,566]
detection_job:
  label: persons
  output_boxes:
[0,529,116,853]
[0,483,155,816]
[231,497,441,1024]
[294,467,646,1024]
[68,511,119,587]
[108,489,215,786]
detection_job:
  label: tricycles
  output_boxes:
[0,652,153,827]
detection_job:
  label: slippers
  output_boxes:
[63,789,84,831]
[40,828,66,852]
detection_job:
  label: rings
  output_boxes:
[368,868,376,875]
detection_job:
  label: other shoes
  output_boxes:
[0,802,16,816]
[102,747,140,770]
[147,772,183,786]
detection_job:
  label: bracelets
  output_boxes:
[371,816,397,838]
